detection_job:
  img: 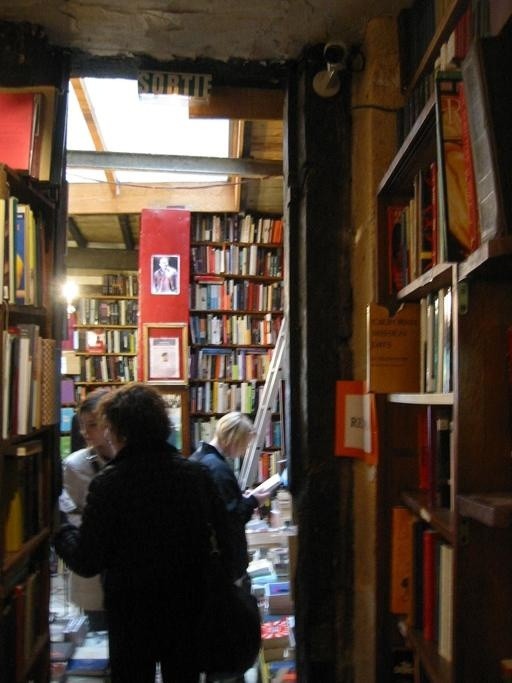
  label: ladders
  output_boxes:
[237,317,288,494]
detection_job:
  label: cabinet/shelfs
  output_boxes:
[68,296,140,386]
[3,68,67,682]
[187,211,284,494]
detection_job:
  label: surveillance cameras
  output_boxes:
[324,38,351,73]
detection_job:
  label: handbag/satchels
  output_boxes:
[192,582,261,681]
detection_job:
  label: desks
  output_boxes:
[374,3,511,681]
